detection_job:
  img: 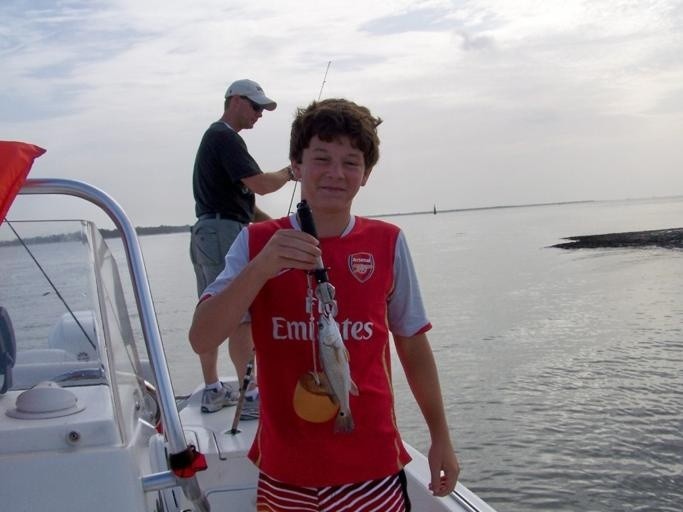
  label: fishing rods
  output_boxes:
[231,60,332,434]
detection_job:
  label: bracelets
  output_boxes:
[287,168,294,181]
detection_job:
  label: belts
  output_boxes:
[197,212,248,225]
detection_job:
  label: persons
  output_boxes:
[188,79,301,421]
[187,99,461,512]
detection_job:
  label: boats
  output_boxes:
[0,170,516,512]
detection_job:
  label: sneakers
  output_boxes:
[201,382,241,415]
[239,393,262,421]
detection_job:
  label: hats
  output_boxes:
[226,79,279,111]
